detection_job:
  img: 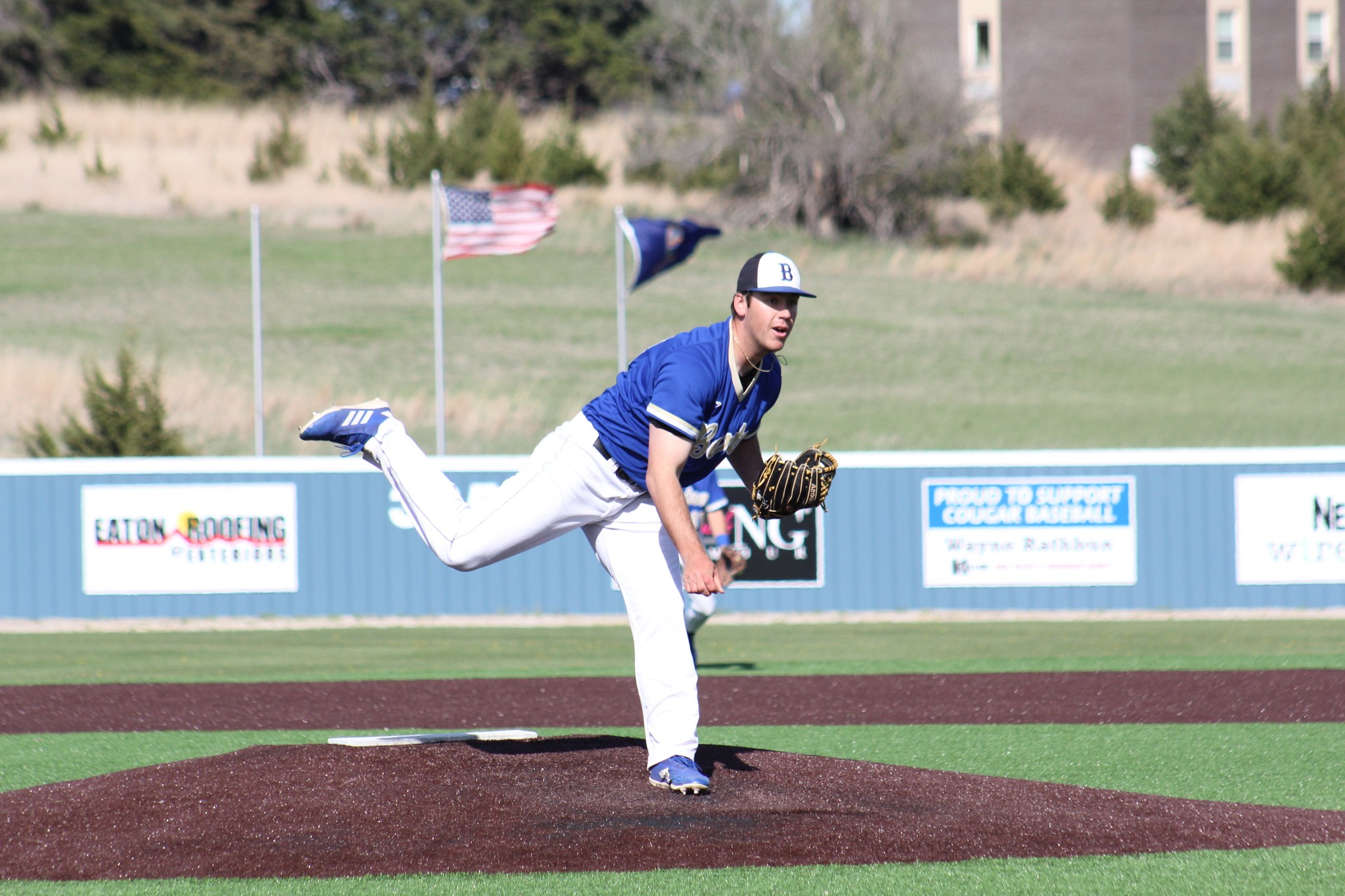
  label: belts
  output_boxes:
[593,437,641,491]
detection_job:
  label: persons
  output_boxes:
[296,251,817,797]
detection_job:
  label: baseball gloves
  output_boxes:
[750,448,838,519]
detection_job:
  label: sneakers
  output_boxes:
[648,754,711,795]
[299,397,394,458]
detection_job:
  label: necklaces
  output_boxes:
[727,323,789,372]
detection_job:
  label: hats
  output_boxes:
[737,250,817,299]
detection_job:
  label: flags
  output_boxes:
[611,214,727,291]
[434,180,562,263]
